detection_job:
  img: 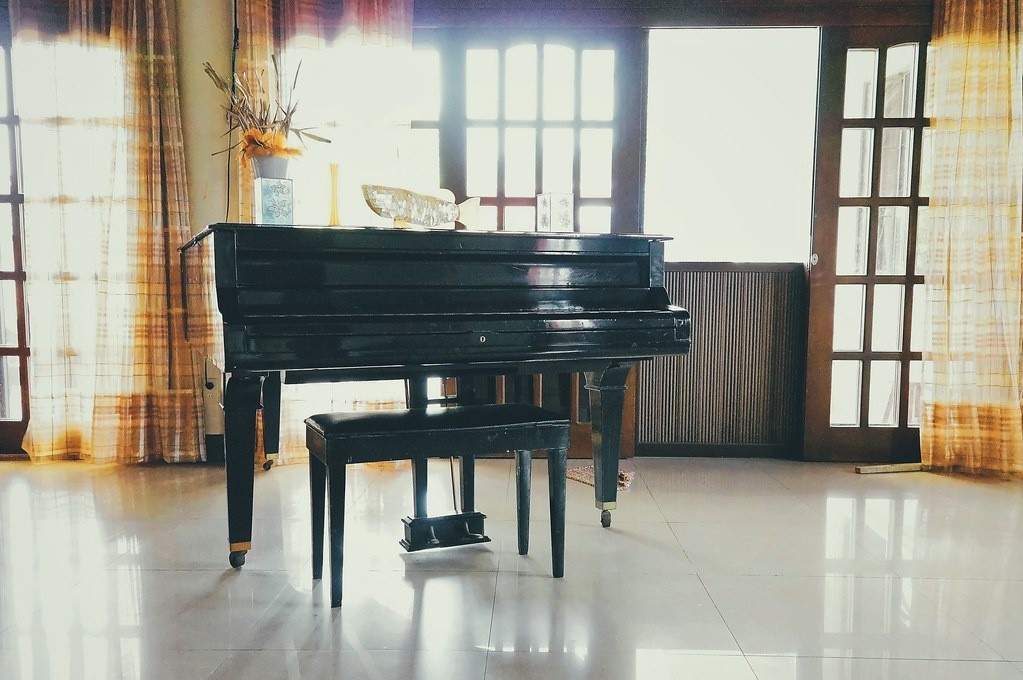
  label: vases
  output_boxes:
[250,156,294,227]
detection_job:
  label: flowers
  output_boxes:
[201,56,334,168]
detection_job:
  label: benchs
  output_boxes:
[303,401,568,609]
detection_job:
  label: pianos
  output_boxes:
[176,222,691,569]
